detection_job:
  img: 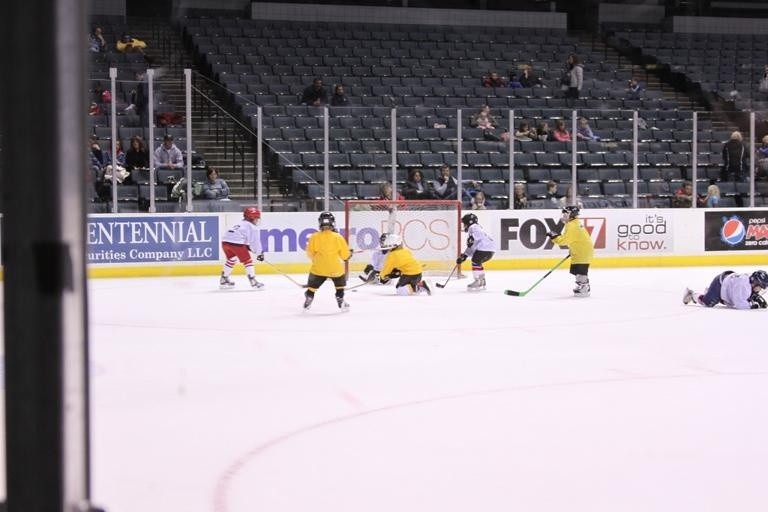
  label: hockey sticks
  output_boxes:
[359,272,394,283]
[251,250,309,288]
[435,263,459,288]
[504,254,570,296]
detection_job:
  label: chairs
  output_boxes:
[85,22,767,212]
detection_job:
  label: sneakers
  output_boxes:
[248,276,264,288]
[219,276,234,285]
[683,290,697,304]
[573,279,590,293]
[410,280,430,295]
[336,296,350,308]
[304,297,311,308]
[467,278,486,287]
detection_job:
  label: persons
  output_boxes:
[379,163,585,209]
[759,73,767,92]
[544,206,594,293]
[672,182,721,208]
[358,232,391,285]
[219,207,265,288]
[682,269,768,310]
[378,234,431,296]
[87,26,236,210]
[455,213,496,288]
[484,55,585,99]
[628,77,641,101]
[722,130,767,181]
[470,103,593,142]
[303,212,353,309]
[299,76,347,107]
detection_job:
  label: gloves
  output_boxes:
[456,253,468,264]
[544,231,560,239]
[373,273,391,286]
[257,255,264,261]
[748,292,768,309]
[467,236,474,247]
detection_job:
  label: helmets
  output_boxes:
[318,212,335,229]
[560,206,579,223]
[462,213,478,232]
[749,270,768,289]
[379,232,402,254]
[244,207,261,226]
